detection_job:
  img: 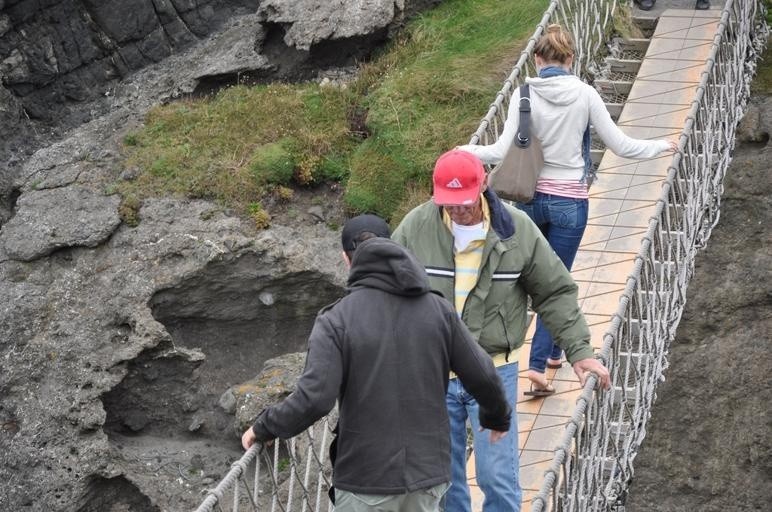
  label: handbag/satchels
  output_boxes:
[487,85,543,204]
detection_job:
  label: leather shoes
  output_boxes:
[695,0,710,9]
[637,0,656,10]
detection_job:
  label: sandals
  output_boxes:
[524,358,562,396]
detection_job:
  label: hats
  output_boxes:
[432,150,485,206]
[342,215,389,250]
